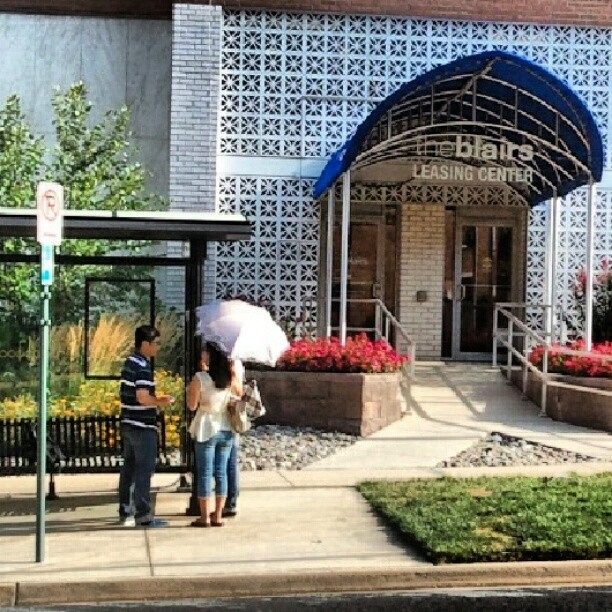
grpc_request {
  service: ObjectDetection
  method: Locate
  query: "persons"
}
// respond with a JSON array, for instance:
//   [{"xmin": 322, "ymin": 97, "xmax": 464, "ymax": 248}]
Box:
[
  {"xmin": 183, "ymin": 343, "xmax": 242, "ymax": 529},
  {"xmin": 209, "ymin": 360, "xmax": 242, "ymax": 517},
  {"xmin": 186, "ymin": 344, "xmax": 213, "ymax": 513},
  {"xmin": 115, "ymin": 324, "xmax": 173, "ymax": 530}
]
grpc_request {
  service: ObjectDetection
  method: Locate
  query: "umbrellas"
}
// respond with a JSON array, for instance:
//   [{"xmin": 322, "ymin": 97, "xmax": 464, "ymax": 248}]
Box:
[{"xmin": 193, "ymin": 298, "xmax": 291, "ymax": 368}]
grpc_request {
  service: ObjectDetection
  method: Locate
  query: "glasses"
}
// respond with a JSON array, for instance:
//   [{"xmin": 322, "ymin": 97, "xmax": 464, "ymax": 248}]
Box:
[{"xmin": 150, "ymin": 341, "xmax": 161, "ymax": 345}]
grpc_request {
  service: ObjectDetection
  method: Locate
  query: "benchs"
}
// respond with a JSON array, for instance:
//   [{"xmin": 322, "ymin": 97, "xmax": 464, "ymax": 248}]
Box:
[{"xmin": 0, "ymin": 416, "xmax": 190, "ymax": 500}]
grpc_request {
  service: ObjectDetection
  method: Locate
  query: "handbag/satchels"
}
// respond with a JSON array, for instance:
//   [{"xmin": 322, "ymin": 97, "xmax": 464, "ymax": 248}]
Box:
[
  {"xmin": 229, "ymin": 401, "xmax": 249, "ymax": 433},
  {"xmin": 242, "ymin": 380, "xmax": 266, "ymax": 421}
]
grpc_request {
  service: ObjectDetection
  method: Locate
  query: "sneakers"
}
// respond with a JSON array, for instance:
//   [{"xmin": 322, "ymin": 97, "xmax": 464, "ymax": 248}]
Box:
[
  {"xmin": 137, "ymin": 520, "xmax": 167, "ymax": 528},
  {"xmin": 121, "ymin": 516, "xmax": 135, "ymax": 526}
]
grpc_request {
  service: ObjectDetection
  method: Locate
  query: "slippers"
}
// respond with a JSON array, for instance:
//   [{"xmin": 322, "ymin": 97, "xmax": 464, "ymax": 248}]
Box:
[
  {"xmin": 210, "ymin": 510, "xmax": 236, "ymax": 516},
  {"xmin": 211, "ymin": 523, "xmax": 223, "ymax": 526},
  {"xmin": 192, "ymin": 518, "xmax": 210, "ymax": 527}
]
